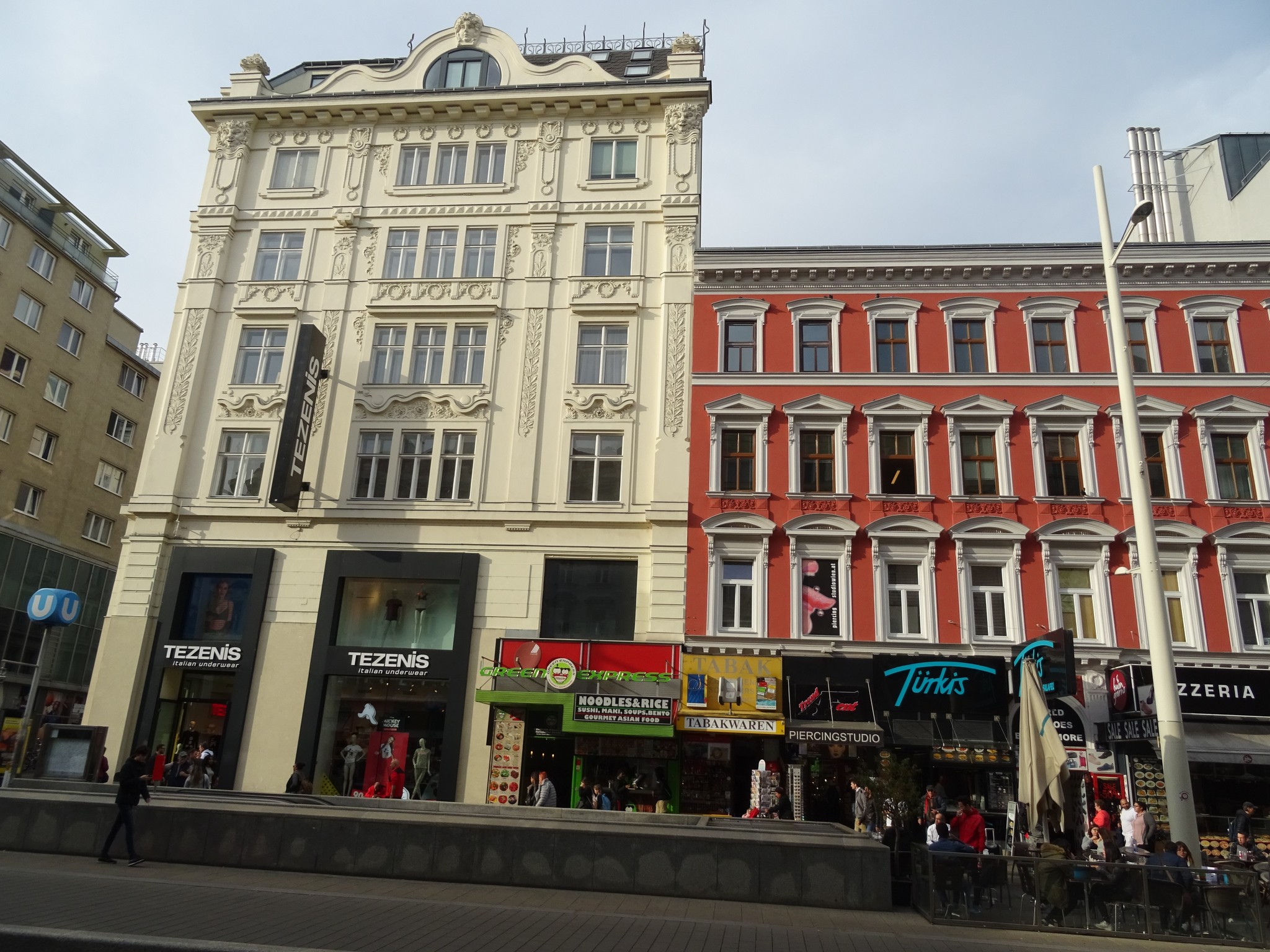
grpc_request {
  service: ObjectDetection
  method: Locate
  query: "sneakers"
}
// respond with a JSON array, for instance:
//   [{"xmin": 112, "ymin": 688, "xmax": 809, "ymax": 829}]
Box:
[
  {"xmin": 1104, "ymin": 923, "xmax": 1116, "ymax": 931},
  {"xmin": 97, "ymin": 857, "xmax": 117, "ymax": 864},
  {"xmin": 128, "ymin": 858, "xmax": 146, "ymax": 866},
  {"xmin": 1095, "ymin": 921, "xmax": 1107, "ymax": 929}
]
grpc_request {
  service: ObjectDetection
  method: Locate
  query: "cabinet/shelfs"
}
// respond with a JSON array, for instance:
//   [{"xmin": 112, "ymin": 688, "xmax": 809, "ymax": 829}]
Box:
[{"xmin": 749, "ymin": 759, "xmax": 805, "ymax": 821}]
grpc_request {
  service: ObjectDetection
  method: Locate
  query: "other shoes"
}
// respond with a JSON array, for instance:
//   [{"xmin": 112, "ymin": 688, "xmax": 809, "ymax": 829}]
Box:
[
  {"xmin": 1042, "ymin": 917, "xmax": 1062, "ymax": 927},
  {"xmin": 1169, "ymin": 926, "xmax": 1194, "ymax": 936}
]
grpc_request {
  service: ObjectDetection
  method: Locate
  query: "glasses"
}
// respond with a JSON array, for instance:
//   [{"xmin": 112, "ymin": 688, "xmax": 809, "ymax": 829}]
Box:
[
  {"xmin": 1092, "ymin": 829, "xmax": 1099, "ymax": 831},
  {"xmin": 927, "ymin": 790, "xmax": 934, "ymax": 792},
  {"xmin": 161, "ymin": 748, "xmax": 165, "ymax": 750},
  {"xmin": 1133, "ymin": 806, "xmax": 1140, "ymax": 808},
  {"xmin": 1178, "ymin": 849, "xmax": 1187, "ymax": 854},
  {"xmin": 786, "ymin": 771, "xmax": 804, "ymax": 821},
  {"xmin": 293, "ymin": 765, "xmax": 295, "ymax": 767}
]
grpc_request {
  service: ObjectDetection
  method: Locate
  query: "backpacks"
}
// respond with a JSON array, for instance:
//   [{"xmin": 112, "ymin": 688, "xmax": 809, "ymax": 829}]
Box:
[
  {"xmin": 586, "ymin": 785, "xmax": 597, "ymax": 809},
  {"xmin": 1229, "ymin": 815, "xmax": 1242, "ymax": 842}
]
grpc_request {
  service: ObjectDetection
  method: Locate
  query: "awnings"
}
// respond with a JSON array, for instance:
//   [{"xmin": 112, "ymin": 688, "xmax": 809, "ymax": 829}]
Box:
[{"xmin": 677, "ymin": 711, "xmax": 884, "ymax": 745}]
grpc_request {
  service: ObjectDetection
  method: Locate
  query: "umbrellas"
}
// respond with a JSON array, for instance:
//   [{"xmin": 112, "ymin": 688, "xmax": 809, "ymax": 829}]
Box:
[{"xmin": 1019, "ymin": 656, "xmax": 1070, "ymax": 842}]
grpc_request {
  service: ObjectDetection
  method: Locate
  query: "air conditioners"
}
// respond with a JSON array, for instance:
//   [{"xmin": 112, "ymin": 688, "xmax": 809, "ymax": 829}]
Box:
[{"xmin": 708, "ymin": 743, "xmax": 730, "ymax": 760}]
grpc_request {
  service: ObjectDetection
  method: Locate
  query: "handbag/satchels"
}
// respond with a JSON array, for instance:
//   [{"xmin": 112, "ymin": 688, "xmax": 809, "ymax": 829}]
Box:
[
  {"xmin": 102, "ymin": 772, "xmax": 109, "ymax": 783},
  {"xmin": 1155, "ymin": 828, "xmax": 1164, "ymax": 841}
]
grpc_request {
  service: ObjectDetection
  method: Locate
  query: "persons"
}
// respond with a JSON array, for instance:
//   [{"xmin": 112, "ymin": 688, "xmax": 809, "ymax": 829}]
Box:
[
  {"xmin": 339, "ymin": 734, "xmax": 368, "ymax": 796},
  {"xmin": 526, "ymin": 771, "xmax": 672, "ymax": 814},
  {"xmin": 378, "ymin": 587, "xmax": 437, "ymax": 647},
  {"xmin": 365, "ymin": 759, "xmax": 404, "ymax": 801},
  {"xmin": 97, "ymin": 740, "xmax": 222, "ymax": 867},
  {"xmin": 285, "ymin": 762, "xmax": 305, "ymax": 796},
  {"xmin": 1020, "ymin": 797, "xmax": 1266, "ymax": 937},
  {"xmin": 377, "ymin": 735, "xmax": 397, "ymax": 794},
  {"xmin": 410, "ymin": 738, "xmax": 432, "ymax": 799},
  {"xmin": 40, "ymin": 700, "xmax": 61, "ymax": 727},
  {"xmin": 204, "ymin": 579, "xmax": 234, "ymax": 635},
  {"xmin": 171, "ymin": 721, "xmax": 200, "ymax": 761},
  {"xmin": 764, "ymin": 786, "xmax": 794, "ymax": 820},
  {"xmin": 852, "ymin": 778, "xmax": 988, "ymax": 913},
  {"xmin": 827, "ymin": 744, "xmax": 845, "ymax": 758}
]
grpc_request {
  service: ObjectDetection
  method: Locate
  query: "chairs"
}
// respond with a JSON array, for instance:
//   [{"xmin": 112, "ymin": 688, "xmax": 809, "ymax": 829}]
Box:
[{"xmin": 935, "ymin": 842, "xmax": 1270, "ymax": 942}]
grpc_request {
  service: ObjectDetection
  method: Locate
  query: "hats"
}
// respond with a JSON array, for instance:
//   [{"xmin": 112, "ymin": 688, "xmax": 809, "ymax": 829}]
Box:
[
  {"xmin": 179, "ymin": 752, "xmax": 187, "ymax": 756},
  {"xmin": 1243, "ymin": 802, "xmax": 1258, "ymax": 808},
  {"xmin": 926, "ymin": 785, "xmax": 934, "ymax": 790}
]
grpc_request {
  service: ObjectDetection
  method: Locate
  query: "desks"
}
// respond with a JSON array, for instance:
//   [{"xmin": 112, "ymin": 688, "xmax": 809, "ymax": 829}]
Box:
[
  {"xmin": 1066, "ymin": 875, "xmax": 1114, "ymax": 929},
  {"xmin": 1192, "ymin": 876, "xmax": 1225, "ymax": 937},
  {"xmin": 602, "ymin": 786, "xmax": 653, "ymax": 812}
]
